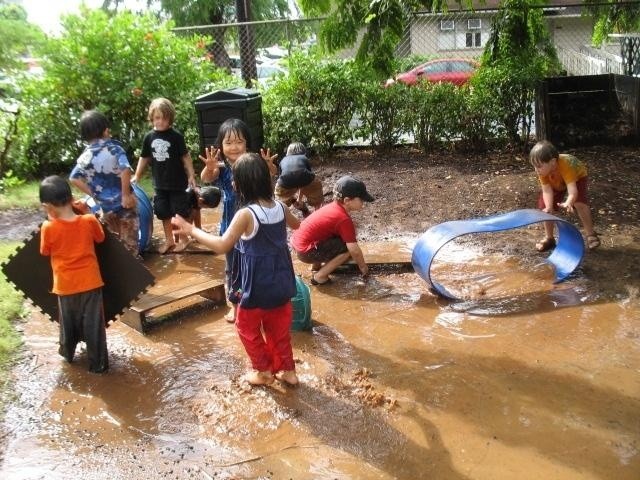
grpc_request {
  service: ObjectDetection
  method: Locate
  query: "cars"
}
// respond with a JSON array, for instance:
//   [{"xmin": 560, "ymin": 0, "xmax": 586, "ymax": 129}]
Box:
[
  {"xmin": 228, "ymin": 35, "xmax": 316, "ymax": 88},
  {"xmin": 383, "ymin": 56, "xmax": 483, "ymax": 89}
]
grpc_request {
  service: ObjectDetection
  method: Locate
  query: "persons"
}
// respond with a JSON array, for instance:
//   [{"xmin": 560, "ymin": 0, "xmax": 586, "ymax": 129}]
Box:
[
  {"xmin": 274, "ymin": 140, "xmax": 323, "ymax": 210},
  {"xmin": 290, "ymin": 174, "xmax": 375, "ymax": 287},
  {"xmin": 198, "ymin": 118, "xmax": 280, "ymax": 324},
  {"xmin": 151, "ymin": 185, "xmax": 221, "ymax": 244},
  {"xmin": 38, "ymin": 174, "xmax": 109, "ymax": 374},
  {"xmin": 527, "ymin": 139, "xmax": 600, "ymax": 251},
  {"xmin": 130, "ymin": 97, "xmax": 198, "ymax": 254},
  {"xmin": 68, "ymin": 108, "xmax": 144, "ymax": 262},
  {"xmin": 170, "ymin": 152, "xmax": 301, "ymax": 388}
]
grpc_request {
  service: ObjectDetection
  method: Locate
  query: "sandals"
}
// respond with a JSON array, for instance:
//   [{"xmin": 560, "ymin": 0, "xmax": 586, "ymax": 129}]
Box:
[
  {"xmin": 586, "ymin": 231, "xmax": 600, "ymax": 249},
  {"xmin": 536, "ymin": 237, "xmax": 555, "ymax": 252},
  {"xmin": 311, "ymin": 273, "xmax": 334, "ymax": 285}
]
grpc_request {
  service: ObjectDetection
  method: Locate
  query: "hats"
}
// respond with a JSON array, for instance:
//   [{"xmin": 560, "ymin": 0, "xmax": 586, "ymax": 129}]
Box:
[{"xmin": 334, "ymin": 176, "xmax": 374, "ymax": 202}]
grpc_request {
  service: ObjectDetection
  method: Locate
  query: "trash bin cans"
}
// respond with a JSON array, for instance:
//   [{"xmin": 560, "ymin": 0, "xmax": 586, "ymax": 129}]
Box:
[{"xmin": 194, "ymin": 88, "xmax": 264, "ymax": 166}]
